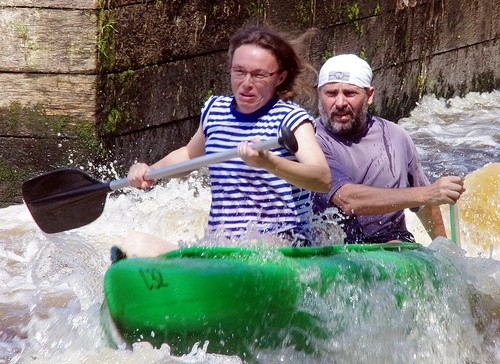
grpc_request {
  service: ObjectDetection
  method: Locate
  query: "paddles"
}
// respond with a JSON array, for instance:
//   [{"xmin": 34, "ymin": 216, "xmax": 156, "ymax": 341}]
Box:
[
  {"xmin": 449, "ymin": 204, "xmax": 461, "ymax": 248},
  {"xmin": 21, "ymin": 125, "xmax": 299, "ymax": 235}
]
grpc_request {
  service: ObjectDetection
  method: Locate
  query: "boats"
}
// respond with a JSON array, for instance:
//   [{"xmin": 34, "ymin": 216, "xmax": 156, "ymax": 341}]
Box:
[{"xmin": 102, "ymin": 241, "xmax": 499, "ymax": 364}]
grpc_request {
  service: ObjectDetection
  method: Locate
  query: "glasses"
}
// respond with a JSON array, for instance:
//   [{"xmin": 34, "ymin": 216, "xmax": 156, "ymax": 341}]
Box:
[{"xmin": 229, "ymin": 66, "xmax": 279, "ymax": 83}]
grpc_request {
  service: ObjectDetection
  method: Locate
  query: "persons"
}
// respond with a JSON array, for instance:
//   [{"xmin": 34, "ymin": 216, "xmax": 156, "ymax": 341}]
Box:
[
  {"xmin": 313, "ymin": 53, "xmax": 468, "ymax": 248},
  {"xmin": 127, "ymin": 23, "xmax": 334, "ymax": 246}
]
